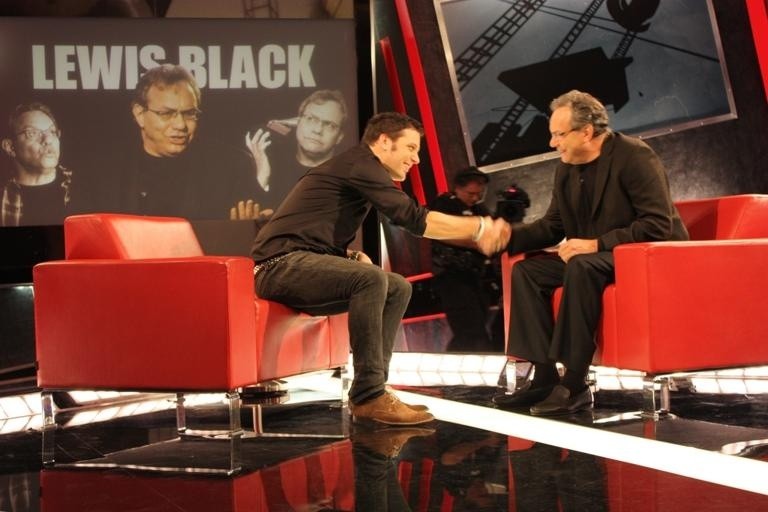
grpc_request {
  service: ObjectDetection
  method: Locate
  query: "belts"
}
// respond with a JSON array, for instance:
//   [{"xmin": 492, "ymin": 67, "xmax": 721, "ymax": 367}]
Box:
[{"xmin": 253, "ymin": 255, "xmax": 283, "ymax": 275}]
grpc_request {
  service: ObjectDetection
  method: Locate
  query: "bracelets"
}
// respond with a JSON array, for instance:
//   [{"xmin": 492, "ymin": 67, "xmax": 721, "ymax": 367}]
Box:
[{"xmin": 472, "ymin": 217, "xmax": 484, "ymax": 243}]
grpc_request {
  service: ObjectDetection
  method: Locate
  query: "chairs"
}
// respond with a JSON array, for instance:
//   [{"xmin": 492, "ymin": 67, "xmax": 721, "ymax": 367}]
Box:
[
  {"xmin": 25, "ymin": 211, "xmax": 358, "ymax": 477},
  {"xmin": 498, "ymin": 190, "xmax": 765, "ymax": 422}
]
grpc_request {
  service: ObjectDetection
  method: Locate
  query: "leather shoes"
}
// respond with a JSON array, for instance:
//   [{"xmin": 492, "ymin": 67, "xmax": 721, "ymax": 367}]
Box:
[
  {"xmin": 348, "ymin": 392, "xmax": 434, "ymax": 426},
  {"xmin": 493, "ymin": 380, "xmax": 553, "ymax": 406},
  {"xmin": 349, "ymin": 427, "xmax": 436, "ymax": 458},
  {"xmin": 530, "ymin": 386, "xmax": 594, "ymax": 416}
]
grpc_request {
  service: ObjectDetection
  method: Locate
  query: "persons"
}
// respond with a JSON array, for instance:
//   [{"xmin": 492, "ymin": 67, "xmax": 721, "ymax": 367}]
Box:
[
  {"xmin": 508, "ymin": 412, "xmax": 607, "ymax": 511},
  {"xmin": 434, "ymin": 383, "xmax": 507, "ymax": 511},
  {"xmin": 76, "ymin": 63, "xmax": 273, "ymax": 219},
  {"xmin": 429, "ymin": 168, "xmax": 496, "ymax": 350},
  {"xmin": 346, "ymin": 411, "xmax": 438, "ymax": 511},
  {"xmin": 0, "ymin": 101, "xmax": 75, "ymax": 225},
  {"xmin": 246, "ymin": 89, "xmax": 347, "ymax": 209},
  {"xmin": 248, "ymin": 112, "xmax": 506, "ymax": 427},
  {"xmin": 489, "ymin": 90, "xmax": 691, "ymax": 416}
]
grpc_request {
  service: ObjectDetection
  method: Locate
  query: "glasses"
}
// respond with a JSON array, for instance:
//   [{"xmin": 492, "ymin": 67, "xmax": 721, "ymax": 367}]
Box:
[
  {"xmin": 146, "ymin": 108, "xmax": 203, "ymax": 120},
  {"xmin": 9, "ymin": 128, "xmax": 61, "ymax": 140}
]
